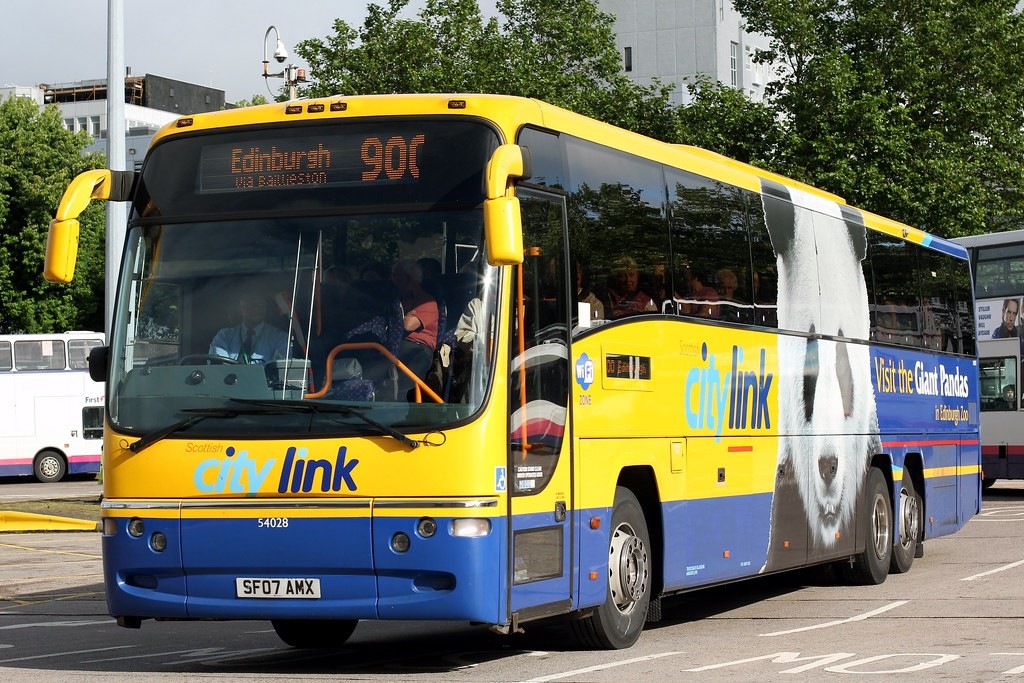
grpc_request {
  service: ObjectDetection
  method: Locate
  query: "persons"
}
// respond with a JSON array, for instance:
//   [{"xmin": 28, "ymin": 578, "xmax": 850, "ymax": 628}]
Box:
[
  {"xmin": 207, "ymin": 280, "xmax": 293, "ymax": 364},
  {"xmin": 453, "ymin": 294, "xmax": 495, "ymax": 354},
  {"xmin": 999, "ymin": 385, "xmax": 1016, "ymax": 409},
  {"xmin": 675, "ymin": 267, "xmax": 720, "ymax": 319},
  {"xmin": 375, "ymin": 259, "xmax": 438, "ymax": 401},
  {"xmin": 575, "ymin": 261, "xmax": 604, "ymax": 320},
  {"xmin": 604, "ymin": 256, "xmax": 657, "ymax": 318},
  {"xmin": 991, "ymin": 299, "xmax": 1019, "ymax": 339},
  {"xmin": 713, "ymin": 268, "xmax": 738, "ymax": 300}
]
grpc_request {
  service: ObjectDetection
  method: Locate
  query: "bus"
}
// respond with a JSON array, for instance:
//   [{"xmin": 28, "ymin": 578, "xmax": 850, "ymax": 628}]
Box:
[
  {"xmin": 45, "ymin": 91, "xmax": 984, "ymax": 650},
  {"xmin": 0, "ymin": 331, "xmax": 106, "ymax": 483},
  {"xmin": 949, "ymin": 229, "xmax": 1024, "ymax": 487}
]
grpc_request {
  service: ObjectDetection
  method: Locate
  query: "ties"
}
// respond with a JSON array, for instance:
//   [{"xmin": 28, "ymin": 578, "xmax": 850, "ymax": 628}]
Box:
[{"xmin": 236, "ymin": 329, "xmax": 255, "ymax": 365}]
